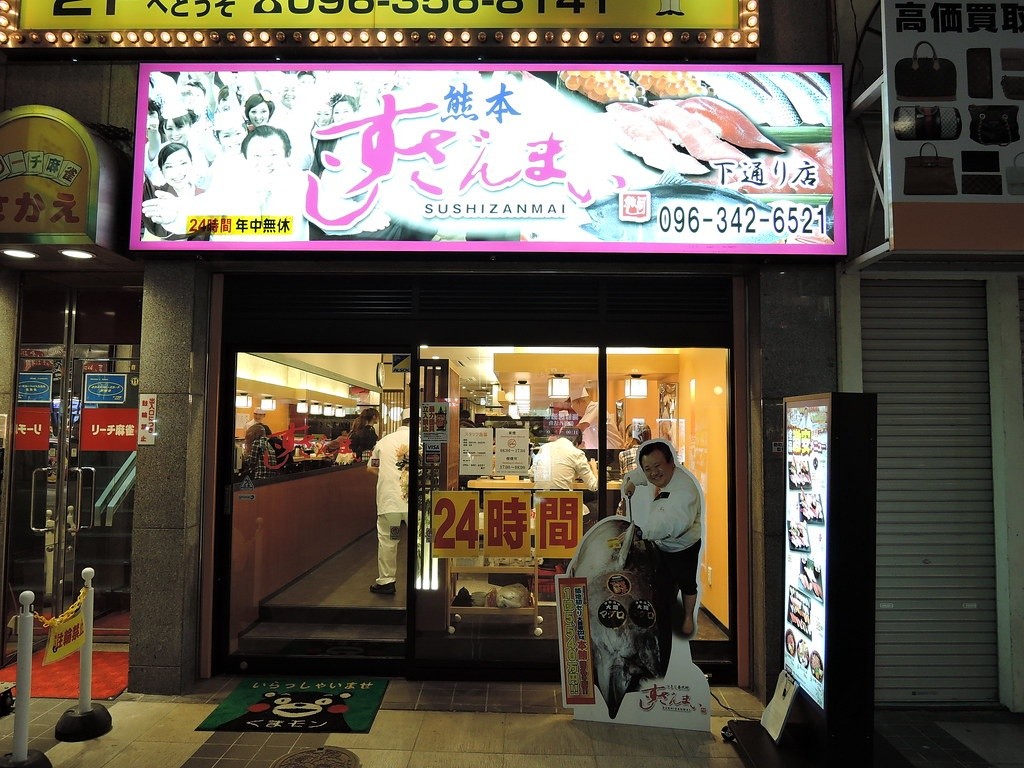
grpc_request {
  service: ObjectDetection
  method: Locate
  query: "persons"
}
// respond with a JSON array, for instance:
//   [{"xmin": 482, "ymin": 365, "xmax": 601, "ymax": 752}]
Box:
[
  {"xmin": 323, "ymin": 430, "xmax": 360, "ymax": 466},
  {"xmin": 547, "ymin": 384, "xmax": 625, "ymax": 465},
  {"xmin": 527, "ymin": 427, "xmax": 599, "ymax": 539},
  {"xmin": 144, "ymin": 70, "xmax": 359, "ymax": 242},
  {"xmin": 243, "ymin": 407, "xmax": 293, "ymax": 479},
  {"xmin": 350, "ymin": 408, "xmax": 380, "ymax": 457},
  {"xmin": 625, "ymin": 442, "xmax": 702, "ymax": 637},
  {"xmin": 367, "ymin": 407, "xmax": 434, "ymax": 595},
  {"xmin": 459, "ymin": 410, "xmax": 477, "ymax": 428},
  {"xmin": 616, "ymin": 423, "xmax": 652, "ymax": 516}
]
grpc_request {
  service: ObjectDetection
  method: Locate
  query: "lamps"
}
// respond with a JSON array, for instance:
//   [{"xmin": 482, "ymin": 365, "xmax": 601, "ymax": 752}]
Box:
[
  {"xmin": 296, "ymin": 400, "xmax": 309, "ymax": 413},
  {"xmin": 513, "ymin": 380, "xmax": 536, "ymax": 400},
  {"xmin": 261, "ymin": 396, "xmax": 276, "ymax": 411},
  {"xmin": 310, "ymin": 402, "xmax": 322, "ymax": 415},
  {"xmin": 548, "ymin": 374, "xmax": 570, "ymax": 399},
  {"xmin": 236, "ymin": 393, "xmax": 252, "ymax": 408},
  {"xmin": 324, "ymin": 404, "xmax": 334, "ymax": 416},
  {"xmin": 625, "ymin": 374, "xmax": 647, "ymax": 398},
  {"xmin": 335, "ymin": 406, "xmax": 346, "ymax": 417}
]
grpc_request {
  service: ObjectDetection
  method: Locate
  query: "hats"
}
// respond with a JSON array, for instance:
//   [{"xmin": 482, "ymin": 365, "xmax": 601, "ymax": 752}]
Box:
[
  {"xmin": 254, "ymin": 407, "xmax": 265, "ymax": 414},
  {"xmin": 403, "ymin": 408, "xmax": 421, "ymax": 420},
  {"xmin": 570, "ymin": 384, "xmax": 588, "ymax": 401}
]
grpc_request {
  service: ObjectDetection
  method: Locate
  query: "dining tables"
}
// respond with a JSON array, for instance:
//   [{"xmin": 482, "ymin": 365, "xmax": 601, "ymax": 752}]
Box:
[{"xmin": 468, "ymin": 475, "xmax": 623, "ymax": 490}]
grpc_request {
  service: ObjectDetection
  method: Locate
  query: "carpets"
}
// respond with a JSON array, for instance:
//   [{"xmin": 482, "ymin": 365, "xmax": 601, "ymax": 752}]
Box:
[
  {"xmin": 0, "ymin": 651, "xmax": 128, "ymax": 701},
  {"xmin": 189, "ymin": 672, "xmax": 391, "ymax": 735}
]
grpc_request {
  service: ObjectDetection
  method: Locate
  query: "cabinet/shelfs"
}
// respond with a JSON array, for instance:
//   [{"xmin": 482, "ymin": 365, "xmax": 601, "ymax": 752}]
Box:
[{"xmin": 445, "ymin": 528, "xmax": 544, "ymax": 637}]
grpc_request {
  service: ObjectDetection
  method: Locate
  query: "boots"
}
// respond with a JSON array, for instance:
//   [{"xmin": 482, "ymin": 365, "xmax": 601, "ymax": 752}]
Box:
[{"xmin": 680, "ymin": 591, "xmax": 697, "ymax": 636}]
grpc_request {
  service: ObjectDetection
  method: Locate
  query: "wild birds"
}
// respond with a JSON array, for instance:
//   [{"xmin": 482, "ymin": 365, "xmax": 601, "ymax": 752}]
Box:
[{"xmin": 566, "ymin": 518, "xmax": 673, "ymax": 720}]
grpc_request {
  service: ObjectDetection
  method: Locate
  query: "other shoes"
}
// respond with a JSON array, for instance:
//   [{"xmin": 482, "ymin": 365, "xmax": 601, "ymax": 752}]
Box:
[{"xmin": 370, "ymin": 580, "xmax": 396, "ymax": 594}]
